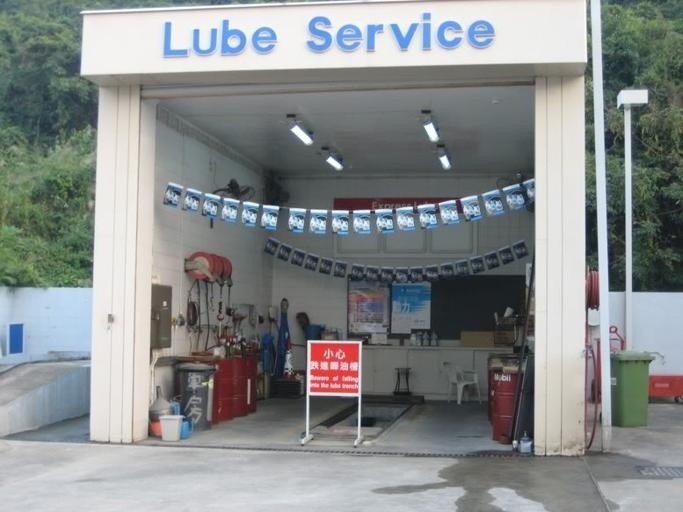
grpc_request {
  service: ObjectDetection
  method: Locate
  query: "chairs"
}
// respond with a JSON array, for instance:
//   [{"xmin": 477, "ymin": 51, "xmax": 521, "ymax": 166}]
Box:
[{"xmin": 442, "ymin": 362, "xmax": 481, "ymax": 405}]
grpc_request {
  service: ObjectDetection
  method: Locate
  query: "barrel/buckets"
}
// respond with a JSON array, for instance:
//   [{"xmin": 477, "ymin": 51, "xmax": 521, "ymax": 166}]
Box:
[
  {"xmin": 232, "ymin": 355, "xmax": 246, "ymax": 417},
  {"xmin": 491, "ymin": 367, "xmax": 522, "ymax": 441},
  {"xmin": 215, "ymin": 359, "xmax": 232, "ymax": 424},
  {"xmin": 180, "ymin": 421, "xmax": 194, "ymax": 438},
  {"xmin": 245, "ymin": 357, "xmax": 257, "ymax": 412}
]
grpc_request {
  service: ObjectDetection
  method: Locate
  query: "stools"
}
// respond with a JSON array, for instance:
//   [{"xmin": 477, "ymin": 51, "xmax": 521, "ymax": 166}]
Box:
[{"xmin": 393, "ymin": 368, "xmax": 411, "ymax": 396}]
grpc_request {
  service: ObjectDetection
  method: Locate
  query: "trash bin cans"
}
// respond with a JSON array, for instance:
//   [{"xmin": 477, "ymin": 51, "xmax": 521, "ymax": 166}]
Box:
[
  {"xmin": 170, "ymin": 394, "xmax": 183, "ymax": 415},
  {"xmin": 612, "ymin": 350, "xmax": 656, "ymax": 428},
  {"xmin": 158, "ymin": 414, "xmax": 183, "ymax": 442}
]
